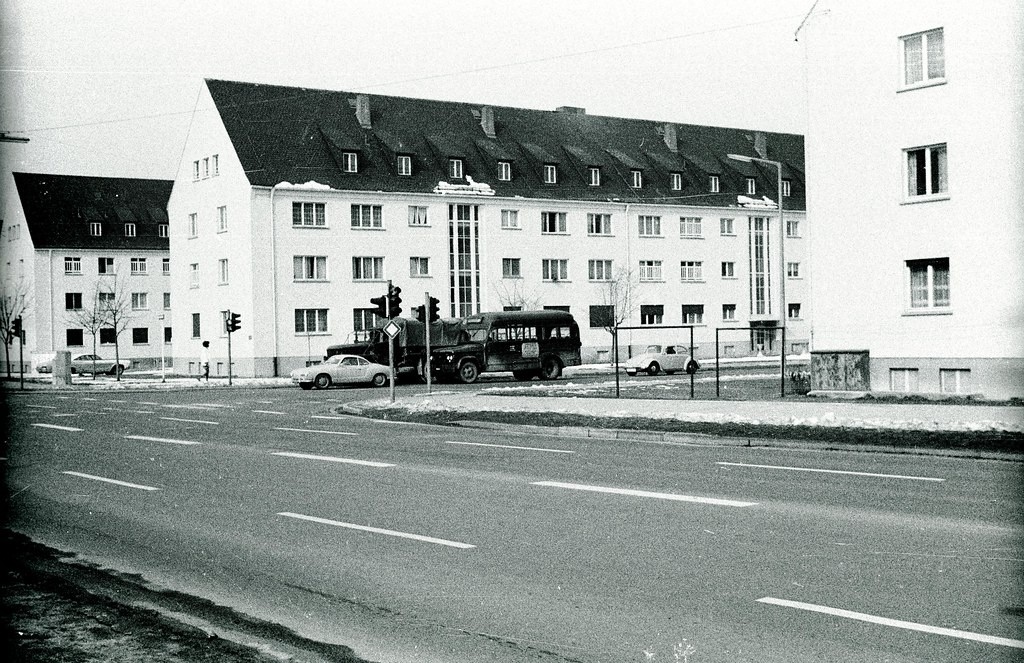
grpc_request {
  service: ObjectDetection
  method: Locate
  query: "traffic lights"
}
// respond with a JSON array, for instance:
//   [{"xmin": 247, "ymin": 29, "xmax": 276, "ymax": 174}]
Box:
[
  {"xmin": 387, "ymin": 284, "xmax": 402, "ymax": 318},
  {"xmin": 371, "ymin": 295, "xmax": 390, "ymax": 318},
  {"xmin": 415, "ymin": 305, "xmax": 426, "ymax": 323},
  {"xmin": 11, "ymin": 318, "xmax": 20, "ymax": 337},
  {"xmin": 230, "ymin": 312, "xmax": 241, "ymax": 332},
  {"xmin": 429, "ymin": 296, "xmax": 440, "ymax": 323}
]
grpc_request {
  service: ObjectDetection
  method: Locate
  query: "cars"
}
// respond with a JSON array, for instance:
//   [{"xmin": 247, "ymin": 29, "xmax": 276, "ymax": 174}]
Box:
[
  {"xmin": 626, "ymin": 342, "xmax": 700, "ymax": 376},
  {"xmin": 291, "ymin": 353, "xmax": 398, "ymax": 390},
  {"xmin": 70, "ymin": 353, "xmax": 133, "ymax": 376},
  {"xmin": 36, "ymin": 359, "xmax": 54, "ymax": 373}
]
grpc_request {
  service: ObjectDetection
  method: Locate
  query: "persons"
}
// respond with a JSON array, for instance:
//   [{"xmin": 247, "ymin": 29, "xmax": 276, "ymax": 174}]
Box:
[{"xmin": 196, "ymin": 341, "xmax": 210, "ymax": 381}]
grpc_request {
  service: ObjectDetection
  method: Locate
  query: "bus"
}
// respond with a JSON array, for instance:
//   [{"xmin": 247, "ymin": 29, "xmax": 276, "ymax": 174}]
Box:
[{"xmin": 431, "ymin": 309, "xmax": 582, "ymax": 385}]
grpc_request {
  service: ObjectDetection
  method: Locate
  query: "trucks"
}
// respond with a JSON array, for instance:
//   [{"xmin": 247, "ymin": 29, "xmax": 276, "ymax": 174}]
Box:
[{"xmin": 322, "ymin": 315, "xmax": 471, "ymax": 371}]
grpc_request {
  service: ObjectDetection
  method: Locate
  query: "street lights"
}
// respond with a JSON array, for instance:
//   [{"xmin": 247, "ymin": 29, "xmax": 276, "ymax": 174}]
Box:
[{"xmin": 727, "ymin": 152, "xmax": 788, "ymax": 361}]
